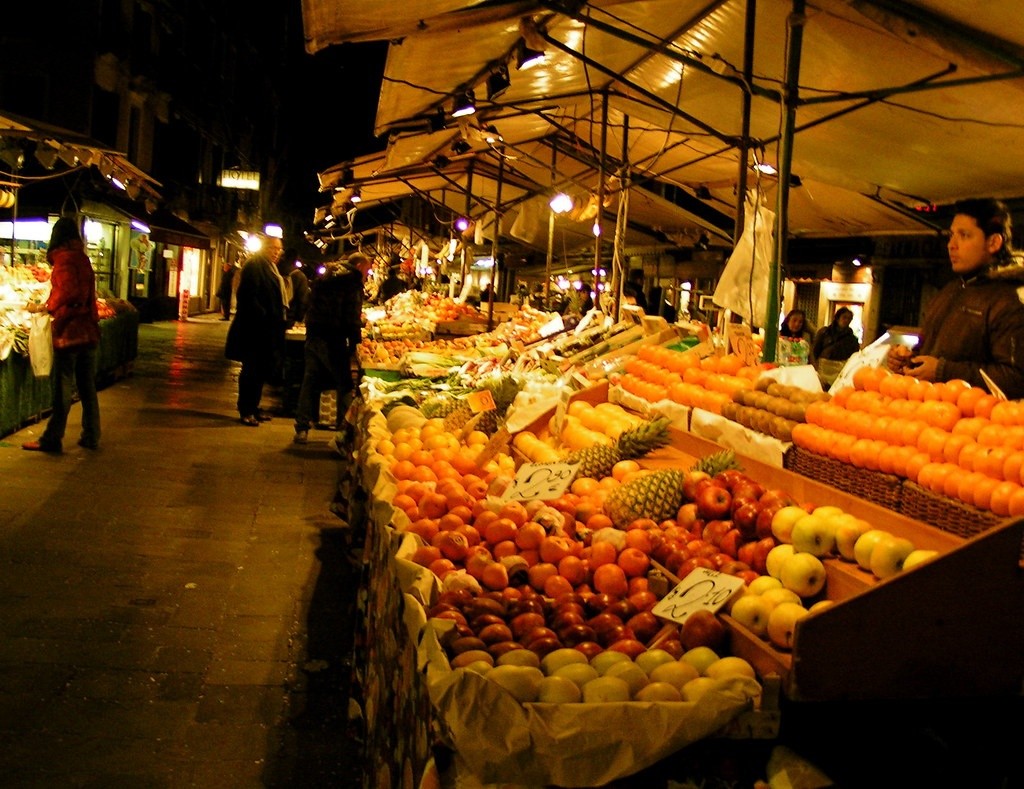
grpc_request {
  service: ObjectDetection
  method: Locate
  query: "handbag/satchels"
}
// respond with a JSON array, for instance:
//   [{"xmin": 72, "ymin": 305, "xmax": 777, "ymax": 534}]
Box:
[{"xmin": 29, "ymin": 311, "xmax": 55, "ymax": 377}]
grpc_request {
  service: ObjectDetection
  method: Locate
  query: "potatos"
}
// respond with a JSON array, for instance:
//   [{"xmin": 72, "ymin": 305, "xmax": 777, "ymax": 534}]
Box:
[{"xmin": 29, "ymin": 266, "xmax": 52, "ymax": 282}]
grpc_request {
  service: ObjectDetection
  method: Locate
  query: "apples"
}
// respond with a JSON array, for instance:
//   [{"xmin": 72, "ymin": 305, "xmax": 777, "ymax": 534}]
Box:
[
  {"xmin": 355, "ymin": 338, "xmax": 473, "ymax": 357},
  {"xmin": 378, "ymin": 298, "xmax": 487, "ymax": 338},
  {"xmin": 427, "ymin": 467, "xmax": 938, "ymax": 703}
]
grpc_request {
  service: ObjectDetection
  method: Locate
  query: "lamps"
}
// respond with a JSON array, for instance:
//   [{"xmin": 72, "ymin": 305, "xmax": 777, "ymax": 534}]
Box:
[
  {"xmin": 57, "ymin": 145, "xmax": 81, "ymax": 168},
  {"xmin": 788, "ymin": 173, "xmax": 802, "ymax": 189},
  {"xmin": 454, "ymin": 141, "xmax": 473, "ymax": 156},
  {"xmin": 432, "ymin": 155, "xmax": 452, "ymax": 169},
  {"xmin": 452, "ymin": 93, "xmax": 475, "ymax": 118},
  {"xmin": 0, "ymin": 146, "xmax": 25, "ymax": 170},
  {"xmin": 126, "ymin": 184, "xmax": 141, "ymax": 199},
  {"xmin": 516, "ymin": 37, "xmax": 545, "ymax": 71},
  {"xmin": 35, "ymin": 141, "xmax": 57, "ymax": 171},
  {"xmin": 486, "ymin": 71, "xmax": 510, "ymax": 101},
  {"xmin": 145, "ymin": 199, "xmax": 158, "ymax": 214},
  {"xmin": 111, "ymin": 170, "xmax": 127, "ymax": 189},
  {"xmin": 431, "ymin": 110, "xmax": 458, "ymax": 134},
  {"xmin": 697, "ymin": 186, "xmax": 711, "ymax": 201},
  {"xmin": 98, "ymin": 158, "xmax": 114, "ymax": 179},
  {"xmin": 76, "ymin": 149, "xmax": 94, "ymax": 168},
  {"xmin": 334, "ymin": 179, "xmax": 346, "ymax": 192}
]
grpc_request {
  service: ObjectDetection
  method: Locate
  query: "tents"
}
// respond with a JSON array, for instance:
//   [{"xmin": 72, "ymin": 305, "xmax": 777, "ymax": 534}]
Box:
[{"xmin": 284, "ymin": 0, "xmax": 1024, "ymax": 256}]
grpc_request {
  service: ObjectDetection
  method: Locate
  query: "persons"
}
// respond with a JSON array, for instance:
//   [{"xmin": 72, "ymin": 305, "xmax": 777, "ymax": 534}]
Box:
[
  {"xmin": 778, "ymin": 307, "xmax": 859, "ymax": 393},
  {"xmin": 21, "ymin": 217, "xmax": 102, "ymax": 455},
  {"xmin": 215, "ymin": 233, "xmax": 409, "ymax": 443},
  {"xmin": 616, "ymin": 269, "xmax": 647, "ymax": 322},
  {"xmin": 888, "ymin": 196, "xmax": 1024, "ymax": 401},
  {"xmin": 481, "ymin": 283, "xmax": 594, "ymax": 318}
]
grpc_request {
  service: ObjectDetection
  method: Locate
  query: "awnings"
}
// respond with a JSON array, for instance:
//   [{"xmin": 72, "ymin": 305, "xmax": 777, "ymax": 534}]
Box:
[
  {"xmin": 150, "ymin": 209, "xmax": 210, "ymax": 250},
  {"xmin": 0, "ymin": 110, "xmax": 163, "ymax": 199}
]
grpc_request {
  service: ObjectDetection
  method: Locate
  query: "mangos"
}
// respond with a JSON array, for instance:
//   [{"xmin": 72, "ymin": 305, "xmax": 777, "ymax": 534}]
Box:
[{"xmin": 96, "ymin": 300, "xmax": 115, "ymax": 319}]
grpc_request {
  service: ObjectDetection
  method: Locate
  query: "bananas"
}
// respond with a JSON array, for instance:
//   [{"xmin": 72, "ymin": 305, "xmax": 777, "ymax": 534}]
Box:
[{"xmin": 0, "ymin": 188, "xmax": 15, "ymax": 208}]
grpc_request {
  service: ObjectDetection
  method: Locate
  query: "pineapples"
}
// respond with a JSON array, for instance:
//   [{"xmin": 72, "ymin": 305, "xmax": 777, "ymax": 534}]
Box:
[
  {"xmin": 559, "ymin": 412, "xmax": 672, "ymax": 480},
  {"xmin": 101, "ymin": 290, "xmax": 133, "ymax": 312},
  {"xmin": 419, "ymin": 377, "xmax": 519, "ymax": 437},
  {"xmin": 602, "ymin": 446, "xmax": 746, "ymax": 528}
]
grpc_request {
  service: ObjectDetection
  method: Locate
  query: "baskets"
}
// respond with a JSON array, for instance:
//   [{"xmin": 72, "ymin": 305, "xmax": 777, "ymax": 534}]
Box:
[
  {"xmin": 901, "ymin": 482, "xmax": 1008, "ymax": 538},
  {"xmin": 785, "ymin": 443, "xmax": 903, "ymax": 513}
]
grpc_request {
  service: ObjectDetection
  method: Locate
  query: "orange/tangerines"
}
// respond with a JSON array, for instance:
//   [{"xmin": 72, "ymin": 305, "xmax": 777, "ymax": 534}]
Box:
[
  {"xmin": 790, "ymin": 365, "xmax": 1024, "ymax": 519},
  {"xmin": 373, "ymin": 400, "xmax": 658, "ymax": 598},
  {"xmin": 619, "ymin": 343, "xmax": 768, "ymax": 417}
]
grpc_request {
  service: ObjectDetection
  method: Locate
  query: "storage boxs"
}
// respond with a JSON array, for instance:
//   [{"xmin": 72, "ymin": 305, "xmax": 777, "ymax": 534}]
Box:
[{"xmin": 350, "ymin": 369, "xmax": 787, "ymax": 789}]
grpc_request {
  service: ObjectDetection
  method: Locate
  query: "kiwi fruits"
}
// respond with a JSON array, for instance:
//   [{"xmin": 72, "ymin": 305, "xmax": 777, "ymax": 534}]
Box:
[{"xmin": 721, "ymin": 377, "xmax": 835, "ymax": 442}]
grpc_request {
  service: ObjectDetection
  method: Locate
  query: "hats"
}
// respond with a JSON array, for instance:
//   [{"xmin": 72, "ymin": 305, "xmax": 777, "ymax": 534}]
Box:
[
  {"xmin": 579, "ymin": 283, "xmax": 592, "ymax": 292},
  {"xmin": 627, "ymin": 268, "xmax": 647, "ymax": 278}
]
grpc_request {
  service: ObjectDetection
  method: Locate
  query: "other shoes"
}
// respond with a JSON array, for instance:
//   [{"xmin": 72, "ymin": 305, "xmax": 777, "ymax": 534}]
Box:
[
  {"xmin": 240, "ymin": 414, "xmax": 259, "ymax": 426},
  {"xmin": 22, "ymin": 440, "xmax": 63, "ymax": 452},
  {"xmin": 219, "ymin": 316, "xmax": 229, "ymax": 321},
  {"xmin": 293, "ymin": 430, "xmax": 307, "ymax": 443},
  {"xmin": 253, "ymin": 410, "xmax": 272, "ymax": 420},
  {"xmin": 77, "ymin": 438, "xmax": 97, "ymax": 450},
  {"xmin": 327, "ymin": 437, "xmax": 346, "ymax": 456}
]
